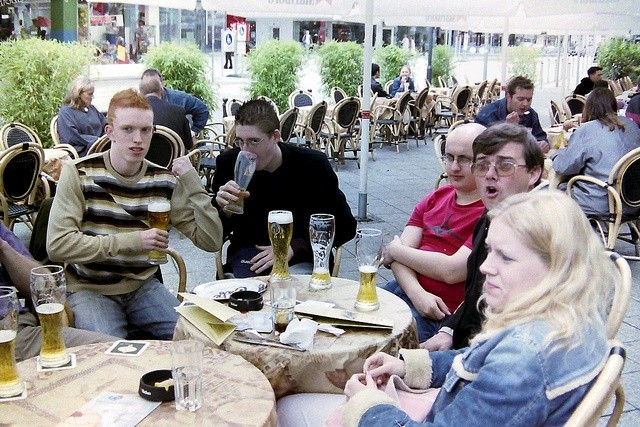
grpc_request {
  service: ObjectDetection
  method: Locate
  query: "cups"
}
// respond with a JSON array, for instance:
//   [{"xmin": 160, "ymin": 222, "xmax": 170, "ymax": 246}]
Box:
[
  {"xmin": 167, "ymin": 339, "xmax": 205, "ymax": 412},
  {"xmin": 29, "ymin": 263, "xmax": 70, "ymax": 367},
  {"xmin": 355, "ymin": 228, "xmax": 383, "ymax": 311},
  {"xmin": 0, "ymin": 285, "xmax": 25, "ymax": 397},
  {"xmin": 308, "ymin": 213, "xmax": 336, "ymax": 290},
  {"xmin": 146, "ymin": 196, "xmax": 171, "ymax": 265},
  {"xmin": 270, "ymin": 285, "xmax": 297, "ymax": 333},
  {"xmin": 223, "ymin": 150, "xmax": 258, "ymax": 215},
  {"xmin": 267, "ymin": 210, "xmax": 293, "ymax": 283}
]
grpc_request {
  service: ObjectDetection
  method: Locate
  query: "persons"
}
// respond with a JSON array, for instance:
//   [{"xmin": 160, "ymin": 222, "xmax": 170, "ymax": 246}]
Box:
[
  {"xmin": 303, "ymin": 30, "xmax": 313, "ymax": 53},
  {"xmin": 419, "ymin": 123, "xmax": 544, "ymax": 351},
  {"xmin": 143, "ymin": 69, "xmax": 208, "ymax": 148},
  {"xmin": 45, "ymin": 87, "xmax": 224, "ymax": 339},
  {"xmin": 400, "ymin": 34, "xmax": 416, "ymax": 50},
  {"xmin": 276, "ymin": 192, "xmax": 624, "ymax": 426},
  {"xmin": 550, "ymin": 88, "xmax": 639, "ymax": 217},
  {"xmin": 211, "ymin": 98, "xmax": 357, "ymax": 278},
  {"xmin": 384, "ymin": 124, "xmax": 487, "ymax": 342},
  {"xmin": 140, "ymin": 76, "xmax": 193, "ymax": 157},
  {"xmin": 56, "ymin": 77, "xmax": 107, "ymax": 157},
  {"xmin": 573, "ymin": 67, "xmax": 609, "ymax": 114},
  {"xmin": 474, "ymin": 75, "xmax": 548, "ymax": 155},
  {"xmin": 391, "ymin": 66, "xmax": 415, "ymax": 96},
  {"xmin": 372, "ymin": 63, "xmax": 389, "ymax": 99},
  {"xmin": 0, "ymin": 222, "xmax": 120, "ymax": 364}
]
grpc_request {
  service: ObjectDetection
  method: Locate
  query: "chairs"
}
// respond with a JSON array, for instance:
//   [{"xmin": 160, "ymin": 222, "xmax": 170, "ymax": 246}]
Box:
[
  {"xmin": 297, "ymin": 100, "xmax": 331, "ymax": 155},
  {"xmin": 602, "ymin": 251, "xmax": 631, "ymax": 339},
  {"xmin": 564, "ymin": 94, "xmax": 587, "ymax": 120},
  {"xmin": 0, "ymin": 142, "xmax": 46, "ymax": 229},
  {"xmin": 194, "ymin": 116, "xmax": 236, "ymax": 194},
  {"xmin": 485, "ymin": 75, "xmax": 501, "ymax": 103},
  {"xmin": 548, "ymin": 99, "xmax": 564, "ymax": 125},
  {"xmin": 450, "ymin": 75, "xmax": 461, "ymax": 86},
  {"xmin": 608, "ymin": 75, "xmax": 635, "ymax": 97},
  {"xmin": 425, "ymin": 78, "xmax": 434, "ymax": 90},
  {"xmin": 566, "ymin": 174, "xmax": 623, "ymax": 251},
  {"xmin": 587, "ymin": 146, "xmax": 640, "ymax": 259},
  {"xmin": 368, "ymin": 89, "xmax": 412, "ymax": 161},
  {"xmin": 564, "ymin": 341, "xmax": 629, "ymax": 426},
  {"xmin": 50, "ymin": 114, "xmax": 79, "ymax": 158},
  {"xmin": 196, "ymin": 122, "xmax": 226, "ymax": 153},
  {"xmin": 40, "ymin": 197, "xmax": 188, "ymax": 300},
  {"xmin": 279, "ymin": 107, "xmax": 300, "ymax": 142},
  {"xmin": 372, "ymin": 91, "xmax": 378, "ymax": 110},
  {"xmin": 433, "ymin": 86, "xmax": 472, "ymax": 134},
  {"xmin": 437, "ymin": 75, "xmax": 448, "ymax": 87},
  {"xmin": 384, "ymin": 79, "xmax": 396, "ymax": 95},
  {"xmin": 332, "ymin": 97, "xmax": 361, "ymax": 167},
  {"xmin": 0, "ymin": 122, "xmax": 43, "ymax": 153},
  {"xmin": 289, "ymin": 89, "xmax": 315, "ymax": 106},
  {"xmin": 87, "ymin": 125, "xmax": 201, "ymax": 176},
  {"xmin": 214, "ymin": 232, "xmax": 343, "ymax": 277},
  {"xmin": 331, "ymin": 86, "xmax": 346, "ymax": 103},
  {"xmin": 471, "ymin": 79, "xmax": 489, "ymax": 118},
  {"xmin": 226, "ymin": 98, "xmax": 242, "ymax": 117},
  {"xmin": 258, "ymin": 94, "xmax": 280, "ymax": 118},
  {"xmin": 401, "ymin": 87, "xmax": 430, "ymax": 148}
]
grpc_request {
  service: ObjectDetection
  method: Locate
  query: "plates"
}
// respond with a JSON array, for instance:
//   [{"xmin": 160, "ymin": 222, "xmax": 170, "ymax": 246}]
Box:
[{"xmin": 192, "ymin": 278, "xmax": 267, "ymax": 302}]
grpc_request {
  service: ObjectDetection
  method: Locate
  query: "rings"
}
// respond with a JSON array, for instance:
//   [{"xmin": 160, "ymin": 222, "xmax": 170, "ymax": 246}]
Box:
[{"xmin": 220, "ymin": 191, "xmax": 224, "ymax": 196}]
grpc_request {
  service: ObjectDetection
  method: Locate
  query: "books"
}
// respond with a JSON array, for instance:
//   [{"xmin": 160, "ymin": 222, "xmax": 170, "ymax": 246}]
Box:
[
  {"xmin": 174, "ymin": 290, "xmax": 237, "ymax": 347},
  {"xmin": 294, "ymin": 299, "xmax": 394, "ymax": 331}
]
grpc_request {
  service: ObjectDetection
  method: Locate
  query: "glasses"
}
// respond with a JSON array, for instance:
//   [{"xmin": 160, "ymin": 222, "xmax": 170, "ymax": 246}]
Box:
[
  {"xmin": 440, "ymin": 156, "xmax": 473, "ymax": 167},
  {"xmin": 471, "ymin": 161, "xmax": 530, "ymax": 176},
  {"xmin": 233, "ymin": 131, "xmax": 273, "ymax": 148}
]
